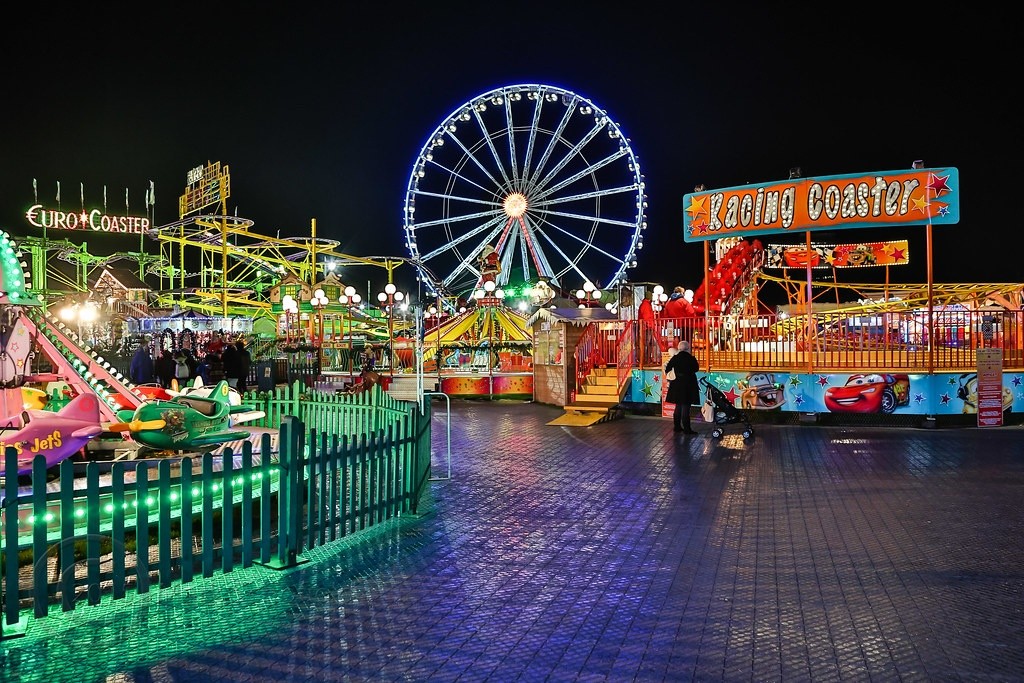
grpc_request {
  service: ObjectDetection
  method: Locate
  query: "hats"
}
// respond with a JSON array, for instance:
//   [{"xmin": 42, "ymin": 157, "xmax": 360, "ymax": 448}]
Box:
[
  {"xmin": 678, "ymin": 341, "xmax": 689, "ymax": 350},
  {"xmin": 646, "ymin": 291, "xmax": 653, "ymax": 302}
]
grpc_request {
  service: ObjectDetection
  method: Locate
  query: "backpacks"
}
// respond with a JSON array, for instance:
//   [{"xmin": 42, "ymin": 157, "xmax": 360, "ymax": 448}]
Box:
[{"xmin": 175, "ymin": 361, "xmax": 189, "ymax": 378}]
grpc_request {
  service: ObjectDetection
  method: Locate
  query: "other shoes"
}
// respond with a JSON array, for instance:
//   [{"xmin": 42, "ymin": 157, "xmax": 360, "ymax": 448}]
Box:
[
  {"xmin": 673, "ymin": 426, "xmax": 684, "ymax": 431},
  {"xmin": 684, "ymin": 427, "xmax": 698, "ymax": 434}
]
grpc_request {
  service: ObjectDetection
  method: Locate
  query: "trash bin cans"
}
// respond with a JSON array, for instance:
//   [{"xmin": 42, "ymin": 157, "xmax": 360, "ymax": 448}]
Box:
[{"xmin": 254, "ymin": 357, "xmax": 278, "ymax": 397}]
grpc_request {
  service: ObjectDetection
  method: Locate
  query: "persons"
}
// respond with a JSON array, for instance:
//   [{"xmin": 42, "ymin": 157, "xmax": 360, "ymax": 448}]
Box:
[
  {"xmin": 638, "ymin": 285, "xmax": 699, "ymax": 364},
  {"xmin": 130, "ymin": 338, "xmax": 250, "ymax": 395},
  {"xmin": 664, "ymin": 341, "xmax": 700, "ymax": 434}
]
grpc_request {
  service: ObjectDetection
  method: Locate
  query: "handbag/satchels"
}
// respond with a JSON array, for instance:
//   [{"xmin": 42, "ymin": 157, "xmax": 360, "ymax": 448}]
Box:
[
  {"xmin": 701, "ymin": 387, "xmax": 714, "ymax": 422},
  {"xmin": 227, "ymin": 378, "xmax": 238, "ymax": 388}
]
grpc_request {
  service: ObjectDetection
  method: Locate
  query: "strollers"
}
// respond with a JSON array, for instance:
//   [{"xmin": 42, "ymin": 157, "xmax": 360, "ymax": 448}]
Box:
[{"xmin": 699, "ymin": 376, "xmax": 755, "ymax": 440}]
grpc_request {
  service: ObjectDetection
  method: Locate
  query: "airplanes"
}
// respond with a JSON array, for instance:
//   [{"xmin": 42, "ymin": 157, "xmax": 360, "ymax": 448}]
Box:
[
  {"xmin": 107, "ymin": 376, "xmax": 264, "ymax": 450},
  {"xmin": 0, "ymin": 393, "xmax": 102, "ymax": 477}
]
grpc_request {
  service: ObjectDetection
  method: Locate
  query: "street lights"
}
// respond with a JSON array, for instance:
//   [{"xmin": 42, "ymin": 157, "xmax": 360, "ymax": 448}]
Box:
[
  {"xmin": 282, "ymin": 295, "xmax": 298, "ymax": 345},
  {"xmin": 652, "ymin": 285, "xmax": 668, "ymax": 318},
  {"xmin": 339, "ymin": 286, "xmax": 361, "ymax": 375},
  {"xmin": 310, "ymin": 289, "xmax": 330, "ymax": 380},
  {"xmin": 578, "ymin": 282, "xmax": 601, "ymax": 308},
  {"xmin": 378, "ymin": 284, "xmax": 403, "ymax": 376},
  {"xmin": 475, "ymin": 281, "xmax": 504, "ymax": 375},
  {"xmin": 426, "ymin": 308, "xmax": 441, "ymax": 328},
  {"xmin": 60, "ymin": 304, "xmax": 98, "ymax": 374}
]
